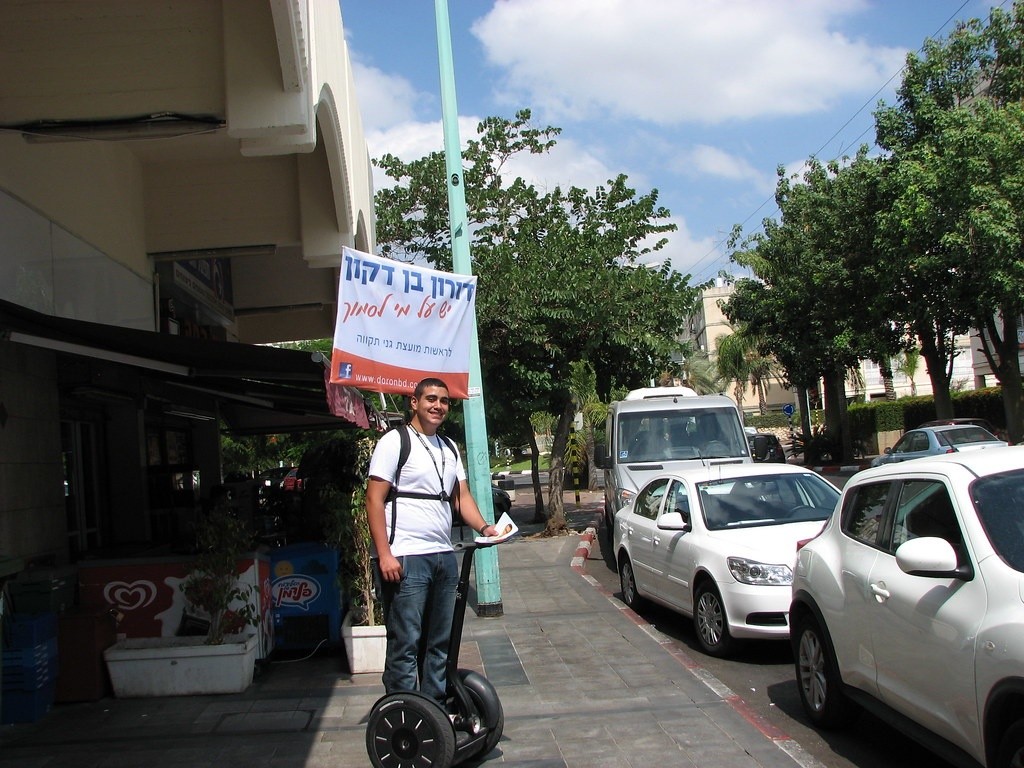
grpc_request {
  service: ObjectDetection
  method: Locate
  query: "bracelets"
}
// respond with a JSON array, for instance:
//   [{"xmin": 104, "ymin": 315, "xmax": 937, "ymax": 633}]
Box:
[{"xmin": 480, "ymin": 525, "xmax": 491, "ymax": 537}]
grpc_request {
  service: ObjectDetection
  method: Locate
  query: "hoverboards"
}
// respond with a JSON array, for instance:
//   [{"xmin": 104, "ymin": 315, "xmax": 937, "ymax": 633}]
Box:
[{"xmin": 365, "ymin": 535, "xmax": 511, "ymax": 768}]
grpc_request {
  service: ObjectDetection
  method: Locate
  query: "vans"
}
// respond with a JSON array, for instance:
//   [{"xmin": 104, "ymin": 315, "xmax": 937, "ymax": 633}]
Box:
[{"xmin": 591, "ymin": 386, "xmax": 755, "ymax": 547}]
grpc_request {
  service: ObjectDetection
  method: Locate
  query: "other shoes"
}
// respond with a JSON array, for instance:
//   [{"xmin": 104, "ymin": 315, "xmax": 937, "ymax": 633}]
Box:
[{"xmin": 448, "ymin": 714, "xmax": 463, "ymax": 724}]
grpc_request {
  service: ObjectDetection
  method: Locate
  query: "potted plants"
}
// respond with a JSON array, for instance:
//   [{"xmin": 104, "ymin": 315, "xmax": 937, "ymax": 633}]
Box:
[
  {"xmin": 104, "ymin": 501, "xmax": 259, "ymax": 693},
  {"xmin": 341, "ymin": 435, "xmax": 390, "ymax": 675}
]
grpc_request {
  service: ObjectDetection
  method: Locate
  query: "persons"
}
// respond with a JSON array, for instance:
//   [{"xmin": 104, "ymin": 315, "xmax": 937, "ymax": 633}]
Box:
[
  {"xmin": 366, "ymin": 379, "xmax": 499, "ymax": 704},
  {"xmin": 488, "ymin": 524, "xmax": 512, "ymax": 541}
]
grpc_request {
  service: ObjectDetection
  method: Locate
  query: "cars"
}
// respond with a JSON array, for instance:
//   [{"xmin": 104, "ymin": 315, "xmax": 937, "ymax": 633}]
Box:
[
  {"xmin": 870, "ymin": 425, "xmax": 1009, "ymax": 468},
  {"xmin": 250, "ymin": 466, "xmax": 301, "ymax": 496},
  {"xmin": 915, "ymin": 418, "xmax": 1002, "ymax": 441},
  {"xmin": 743, "ymin": 425, "xmax": 787, "ymax": 464},
  {"xmin": 787, "ymin": 444, "xmax": 1024, "ymax": 768},
  {"xmin": 612, "ymin": 463, "xmax": 849, "ymax": 654}
]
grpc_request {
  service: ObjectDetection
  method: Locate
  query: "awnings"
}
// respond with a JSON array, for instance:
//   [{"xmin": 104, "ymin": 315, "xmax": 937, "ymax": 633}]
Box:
[{"xmin": 0, "ymin": 312, "xmax": 369, "ymax": 433}]
